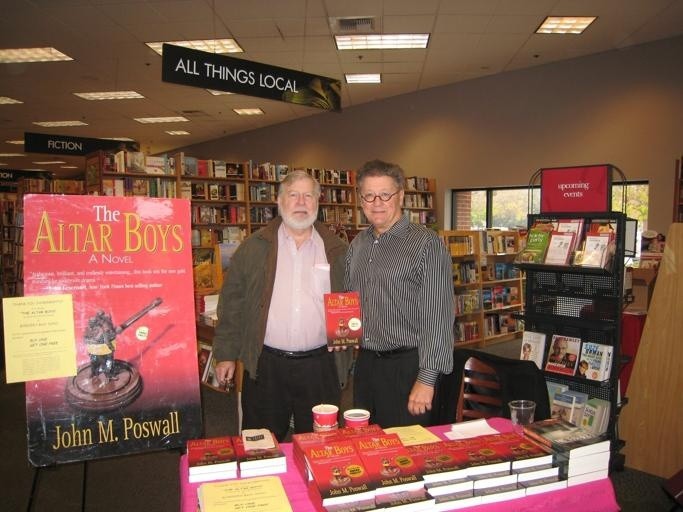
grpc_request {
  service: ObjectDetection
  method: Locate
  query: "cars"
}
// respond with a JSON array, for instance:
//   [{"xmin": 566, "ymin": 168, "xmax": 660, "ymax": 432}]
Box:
[
  {"xmin": 507, "ymin": 400, "xmax": 537, "ymax": 426},
  {"xmin": 311, "ymin": 404, "xmax": 340, "ymax": 427},
  {"xmin": 342, "ymin": 408, "xmax": 372, "ymax": 431}
]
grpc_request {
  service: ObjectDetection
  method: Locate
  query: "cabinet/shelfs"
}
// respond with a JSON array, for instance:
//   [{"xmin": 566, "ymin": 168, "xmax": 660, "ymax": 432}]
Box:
[
  {"xmin": 510, "ymin": 212, "xmax": 626, "ymax": 476},
  {"xmin": 1, "ymin": 149, "xmax": 529, "ymax": 349}
]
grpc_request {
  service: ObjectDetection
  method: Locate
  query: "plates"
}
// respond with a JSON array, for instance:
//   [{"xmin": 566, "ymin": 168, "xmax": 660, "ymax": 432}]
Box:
[{"xmin": 510, "ymin": 212, "xmax": 626, "ymax": 476}]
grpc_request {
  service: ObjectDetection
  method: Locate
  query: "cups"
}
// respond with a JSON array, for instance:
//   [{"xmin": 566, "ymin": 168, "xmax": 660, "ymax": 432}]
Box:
[{"xmin": 359, "ymin": 189, "xmax": 401, "ymax": 203}]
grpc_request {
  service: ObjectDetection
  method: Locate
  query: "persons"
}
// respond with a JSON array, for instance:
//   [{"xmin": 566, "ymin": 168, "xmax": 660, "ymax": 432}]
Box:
[
  {"xmin": 212, "ymin": 170, "xmax": 349, "ymax": 443},
  {"xmin": 340, "ymin": 160, "xmax": 456, "ymax": 432}
]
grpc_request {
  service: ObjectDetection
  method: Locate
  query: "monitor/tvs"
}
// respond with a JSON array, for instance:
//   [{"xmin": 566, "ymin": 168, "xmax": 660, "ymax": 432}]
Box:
[{"xmin": 513, "ymin": 220, "xmax": 615, "ymax": 434}]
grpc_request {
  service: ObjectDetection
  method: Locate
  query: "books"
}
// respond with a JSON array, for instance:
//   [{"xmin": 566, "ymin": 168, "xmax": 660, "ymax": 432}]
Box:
[
  {"xmin": 184, "ymin": 156, "xmax": 289, "ymax": 286},
  {"xmin": 304, "ymin": 168, "xmax": 369, "ymax": 224},
  {"xmin": 185, "ymin": 416, "xmax": 612, "ymax": 512},
  {"xmin": 198, "ymin": 342, "xmax": 233, "ymax": 392},
  {"xmin": 4, "ymin": 193, "xmax": 24, "ymax": 297},
  {"xmin": 447, "ymin": 232, "xmax": 514, "ymax": 342},
  {"xmin": 23, "ymin": 179, "xmax": 83, "ymax": 194},
  {"xmin": 513, "ymin": 220, "xmax": 615, "ymax": 434},
  {"xmin": 196, "ymin": 287, "xmax": 220, "ymax": 325},
  {"xmin": 85, "ymin": 152, "xmax": 177, "ymax": 199}
]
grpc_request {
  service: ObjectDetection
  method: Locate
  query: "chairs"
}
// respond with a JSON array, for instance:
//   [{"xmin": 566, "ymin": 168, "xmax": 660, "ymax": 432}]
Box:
[{"xmin": 454, "ymin": 357, "xmax": 504, "ymax": 423}]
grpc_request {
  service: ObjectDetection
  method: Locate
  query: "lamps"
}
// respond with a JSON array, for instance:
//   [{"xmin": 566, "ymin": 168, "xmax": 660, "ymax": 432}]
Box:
[{"xmin": 454, "ymin": 357, "xmax": 504, "ymax": 423}]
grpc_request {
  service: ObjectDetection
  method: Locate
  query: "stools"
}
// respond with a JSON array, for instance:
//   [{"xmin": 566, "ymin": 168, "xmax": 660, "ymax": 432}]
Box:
[
  {"xmin": 263, "ymin": 345, "xmax": 327, "ymax": 360},
  {"xmin": 374, "ymin": 347, "xmax": 403, "ymax": 360}
]
